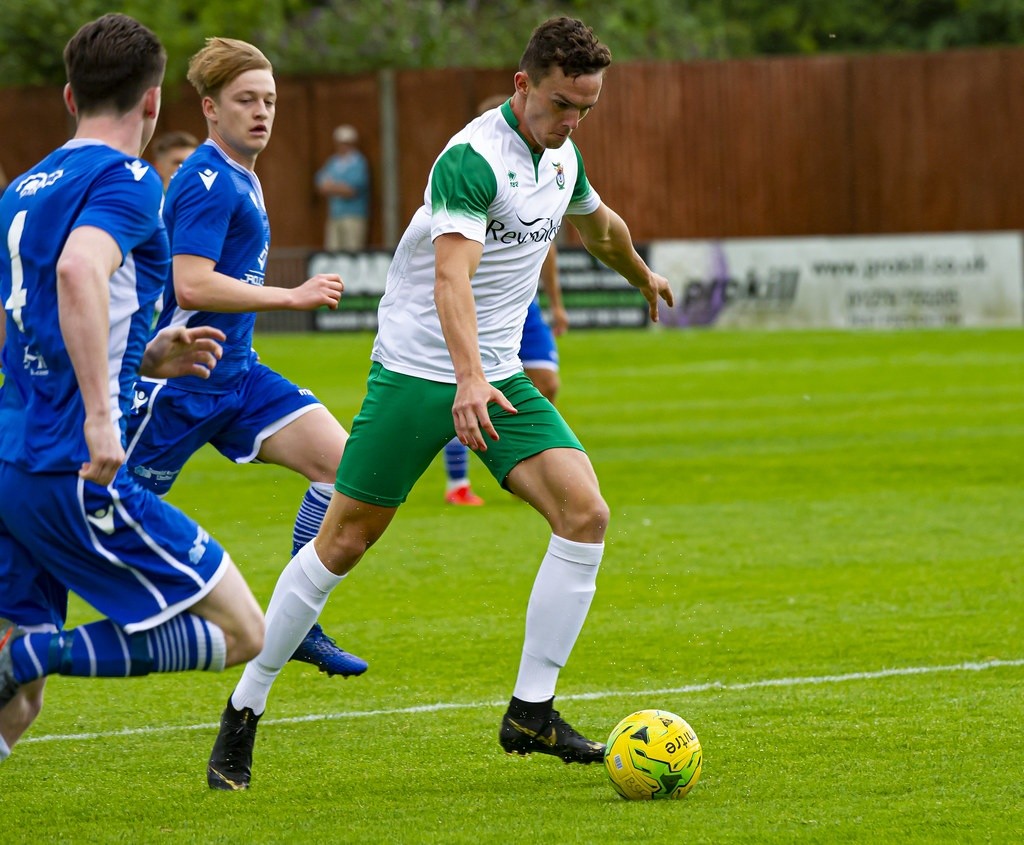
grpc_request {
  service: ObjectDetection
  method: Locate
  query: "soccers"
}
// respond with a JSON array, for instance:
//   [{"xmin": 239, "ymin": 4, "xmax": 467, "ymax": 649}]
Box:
[{"xmin": 604, "ymin": 708, "xmax": 705, "ymax": 802}]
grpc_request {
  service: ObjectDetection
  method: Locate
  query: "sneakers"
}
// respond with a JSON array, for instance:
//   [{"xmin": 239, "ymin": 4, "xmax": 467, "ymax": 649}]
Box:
[
  {"xmin": 446, "ymin": 478, "xmax": 482, "ymax": 505},
  {"xmin": 0, "ymin": 616, "xmax": 26, "ymax": 712},
  {"xmin": 499, "ymin": 709, "xmax": 606, "ymax": 766},
  {"xmin": 288, "ymin": 622, "xmax": 369, "ymax": 681},
  {"xmin": 207, "ymin": 709, "xmax": 264, "ymax": 793}
]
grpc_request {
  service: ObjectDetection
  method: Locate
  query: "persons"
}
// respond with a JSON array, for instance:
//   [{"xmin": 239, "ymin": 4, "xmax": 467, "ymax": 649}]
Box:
[
  {"xmin": 446, "ymin": 94, "xmax": 567, "ymax": 504},
  {"xmin": 205, "ymin": 16, "xmax": 672, "ymax": 799},
  {"xmin": 153, "ymin": 130, "xmax": 199, "ymax": 194},
  {"xmin": 122, "ymin": 32, "xmax": 368, "ymax": 680},
  {"xmin": 0, "ymin": 11, "xmax": 268, "ymax": 766},
  {"xmin": 314, "ymin": 124, "xmax": 370, "ymax": 254}
]
